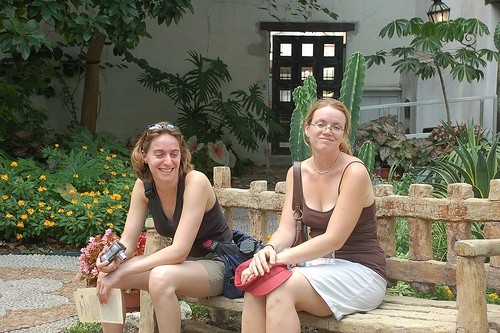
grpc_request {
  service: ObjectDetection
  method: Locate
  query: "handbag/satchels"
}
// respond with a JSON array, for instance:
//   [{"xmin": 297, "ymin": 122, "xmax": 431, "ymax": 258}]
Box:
[
  {"xmin": 217, "ymin": 240, "xmax": 243, "ymax": 299},
  {"xmin": 292, "ymin": 160, "xmax": 303, "ymax": 265}
]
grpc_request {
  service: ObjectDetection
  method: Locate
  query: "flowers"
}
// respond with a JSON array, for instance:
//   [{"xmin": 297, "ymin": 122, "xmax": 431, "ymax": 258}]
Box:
[{"xmin": 75, "ymin": 224, "xmax": 147, "ymax": 288}]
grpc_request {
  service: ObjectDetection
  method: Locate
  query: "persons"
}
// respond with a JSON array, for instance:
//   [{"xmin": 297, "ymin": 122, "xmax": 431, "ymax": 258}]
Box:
[
  {"xmin": 95, "ymin": 121, "xmax": 244, "ymax": 333},
  {"xmin": 241, "ymin": 98, "xmax": 387, "ymax": 333}
]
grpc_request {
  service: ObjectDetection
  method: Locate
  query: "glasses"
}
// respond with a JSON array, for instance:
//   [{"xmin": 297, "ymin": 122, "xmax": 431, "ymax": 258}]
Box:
[
  {"xmin": 141, "ymin": 124, "xmax": 178, "ymax": 151},
  {"xmin": 309, "ymin": 123, "xmax": 345, "ymax": 135}
]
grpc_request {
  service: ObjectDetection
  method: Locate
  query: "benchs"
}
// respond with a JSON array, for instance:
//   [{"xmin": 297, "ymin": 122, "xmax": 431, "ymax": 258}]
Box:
[{"xmin": 138, "ymin": 157, "xmax": 500, "ymax": 333}]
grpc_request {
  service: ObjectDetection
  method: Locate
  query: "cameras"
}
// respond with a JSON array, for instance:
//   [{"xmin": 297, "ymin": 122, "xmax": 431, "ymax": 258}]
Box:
[{"xmin": 101, "ymin": 241, "xmax": 128, "ymax": 265}]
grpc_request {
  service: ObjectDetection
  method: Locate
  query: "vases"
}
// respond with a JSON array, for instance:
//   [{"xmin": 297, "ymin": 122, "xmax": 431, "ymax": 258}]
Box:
[{"xmin": 71, "ymin": 286, "xmax": 141, "ymax": 333}]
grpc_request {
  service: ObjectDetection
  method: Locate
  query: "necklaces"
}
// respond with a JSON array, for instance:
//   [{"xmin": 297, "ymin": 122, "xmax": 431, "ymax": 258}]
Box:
[{"xmin": 310, "ymin": 151, "xmax": 341, "ymax": 174}]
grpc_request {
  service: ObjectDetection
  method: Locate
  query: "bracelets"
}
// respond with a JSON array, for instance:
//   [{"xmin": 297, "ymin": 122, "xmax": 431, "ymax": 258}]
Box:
[{"xmin": 264, "ymin": 244, "xmax": 274, "ymax": 250}]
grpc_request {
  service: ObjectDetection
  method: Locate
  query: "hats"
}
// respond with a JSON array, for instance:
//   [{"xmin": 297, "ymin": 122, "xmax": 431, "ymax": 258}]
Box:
[{"xmin": 233, "ymin": 257, "xmax": 292, "ymax": 295}]
